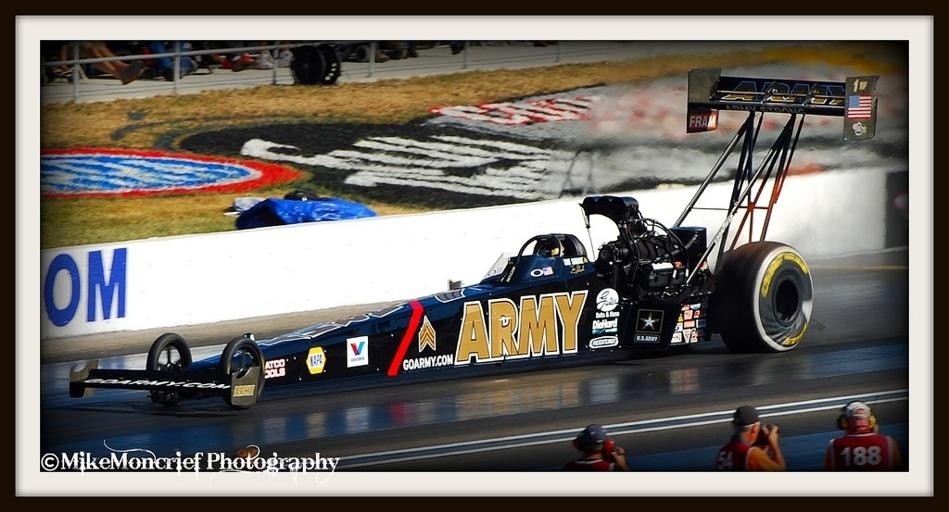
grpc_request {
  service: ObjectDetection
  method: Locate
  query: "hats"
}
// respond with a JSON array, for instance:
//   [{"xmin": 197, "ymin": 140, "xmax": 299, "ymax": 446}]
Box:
[
  {"xmin": 577, "ymin": 424, "xmax": 606, "ymax": 443},
  {"xmin": 734, "ymin": 406, "xmax": 759, "ymax": 425},
  {"xmin": 845, "ymin": 402, "xmax": 871, "ymax": 419}
]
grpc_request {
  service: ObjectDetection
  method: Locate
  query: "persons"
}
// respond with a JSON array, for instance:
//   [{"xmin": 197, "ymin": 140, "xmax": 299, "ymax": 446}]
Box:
[
  {"xmin": 562, "ymin": 424, "xmax": 631, "ymax": 471},
  {"xmin": 550, "ymin": 237, "xmax": 560, "ymax": 257},
  {"xmin": 823, "ymin": 400, "xmax": 901, "ymax": 472},
  {"xmin": 40, "ymin": 41, "xmax": 390, "ymax": 84},
  {"xmin": 712, "ymin": 406, "xmax": 789, "ymax": 471}
]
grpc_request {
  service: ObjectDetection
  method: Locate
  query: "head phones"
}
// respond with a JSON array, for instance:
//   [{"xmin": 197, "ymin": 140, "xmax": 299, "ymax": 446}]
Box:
[
  {"xmin": 836, "ymin": 399, "xmax": 877, "ymax": 429},
  {"xmin": 573, "ymin": 423, "xmax": 614, "ymax": 455}
]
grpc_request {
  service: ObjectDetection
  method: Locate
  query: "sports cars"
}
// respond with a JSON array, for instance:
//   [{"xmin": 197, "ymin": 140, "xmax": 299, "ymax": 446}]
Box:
[{"xmin": 69, "ymin": 68, "xmax": 880, "ymax": 410}]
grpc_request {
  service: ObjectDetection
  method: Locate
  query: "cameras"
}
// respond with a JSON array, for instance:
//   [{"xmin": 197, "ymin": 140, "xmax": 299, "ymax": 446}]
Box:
[{"xmin": 760, "ymin": 423, "xmax": 779, "ymax": 442}]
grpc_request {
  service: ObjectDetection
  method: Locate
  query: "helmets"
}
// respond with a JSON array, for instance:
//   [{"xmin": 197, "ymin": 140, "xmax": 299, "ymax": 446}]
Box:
[{"xmin": 537, "ymin": 239, "xmax": 567, "ymax": 258}]
